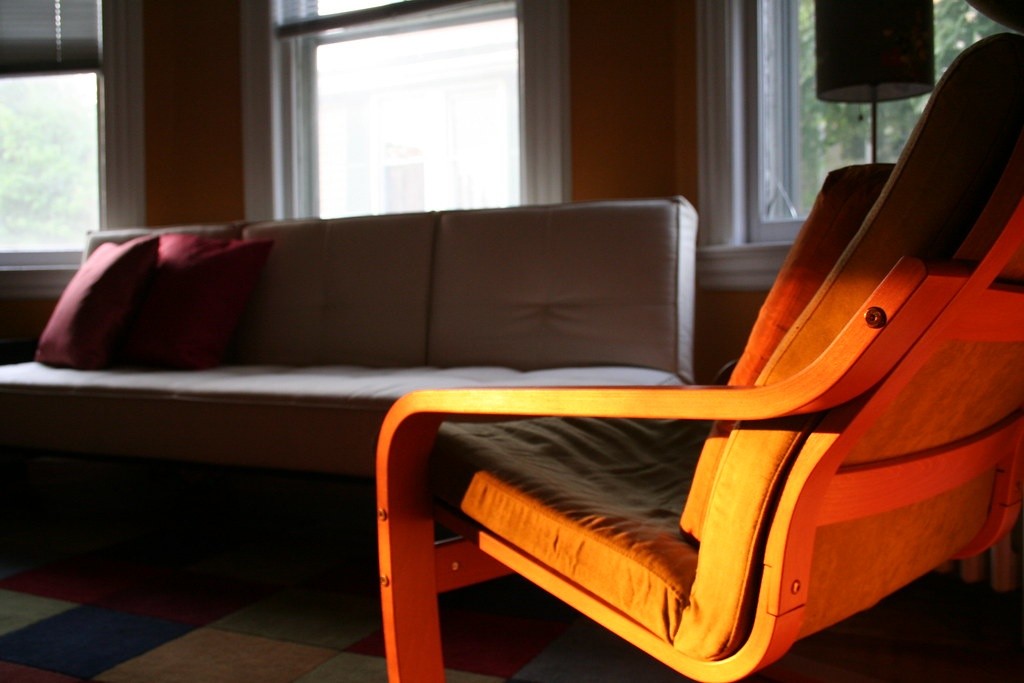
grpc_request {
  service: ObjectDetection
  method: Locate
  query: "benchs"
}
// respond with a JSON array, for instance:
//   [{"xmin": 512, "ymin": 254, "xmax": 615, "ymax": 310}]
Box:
[{"xmin": 0, "ymin": 194, "xmax": 699, "ymax": 481}]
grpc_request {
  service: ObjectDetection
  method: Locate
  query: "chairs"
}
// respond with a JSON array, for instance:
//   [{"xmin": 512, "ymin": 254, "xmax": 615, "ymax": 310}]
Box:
[{"xmin": 376, "ymin": 0, "xmax": 1024, "ymax": 683}]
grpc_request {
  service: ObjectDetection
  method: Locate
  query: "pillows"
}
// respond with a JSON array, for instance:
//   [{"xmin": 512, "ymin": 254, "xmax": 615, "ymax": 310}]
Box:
[
  {"xmin": 122, "ymin": 234, "xmax": 276, "ymax": 371},
  {"xmin": 36, "ymin": 234, "xmax": 160, "ymax": 370}
]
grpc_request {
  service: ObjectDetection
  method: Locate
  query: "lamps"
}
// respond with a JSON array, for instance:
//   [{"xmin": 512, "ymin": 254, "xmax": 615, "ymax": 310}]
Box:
[{"xmin": 814, "ymin": 0, "xmax": 935, "ymax": 165}]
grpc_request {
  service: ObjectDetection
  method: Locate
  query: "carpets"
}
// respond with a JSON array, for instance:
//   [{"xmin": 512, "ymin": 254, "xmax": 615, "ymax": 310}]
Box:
[{"xmin": 0, "ymin": 540, "xmax": 816, "ymax": 683}]
